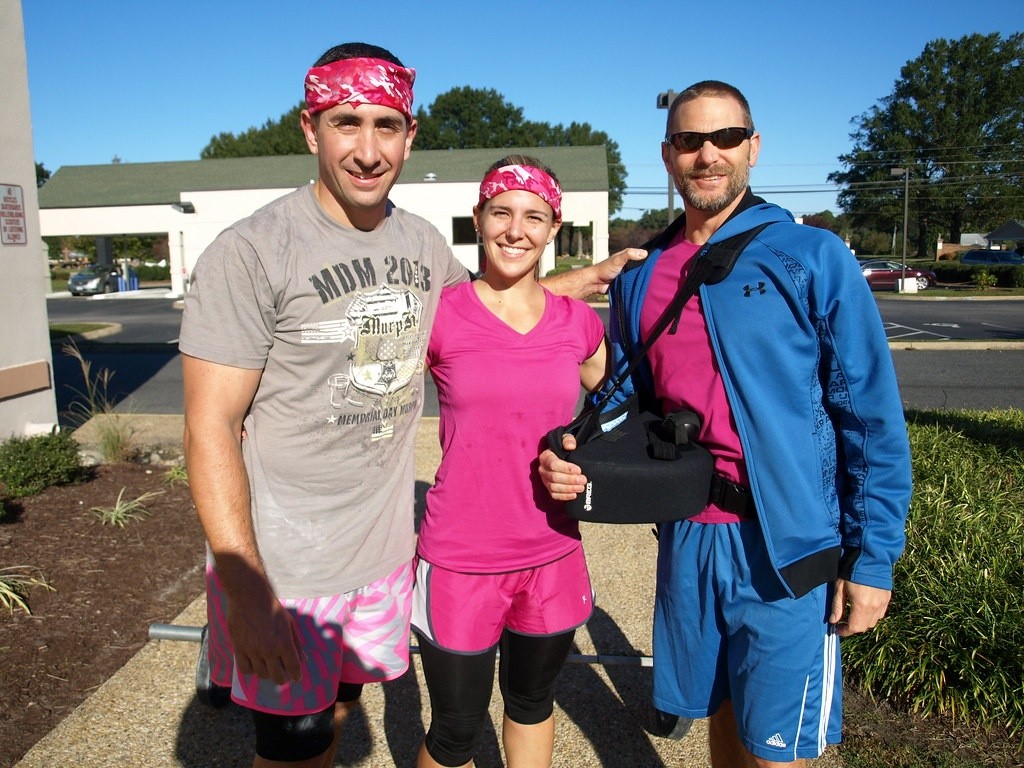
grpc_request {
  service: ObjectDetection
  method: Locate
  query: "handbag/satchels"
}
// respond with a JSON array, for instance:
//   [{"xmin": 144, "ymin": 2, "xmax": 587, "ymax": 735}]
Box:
[{"xmin": 547, "ymin": 389, "xmax": 714, "ymax": 524}]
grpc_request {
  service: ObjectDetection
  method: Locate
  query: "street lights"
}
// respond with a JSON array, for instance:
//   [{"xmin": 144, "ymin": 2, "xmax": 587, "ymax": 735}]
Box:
[
  {"xmin": 657, "ymin": 88, "xmax": 682, "ymax": 229},
  {"xmin": 889, "ymin": 167, "xmax": 910, "ymax": 293}
]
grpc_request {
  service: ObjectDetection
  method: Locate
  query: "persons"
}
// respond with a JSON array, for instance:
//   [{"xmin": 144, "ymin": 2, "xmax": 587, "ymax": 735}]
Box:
[
  {"xmin": 416, "ymin": 152, "xmax": 605, "ymax": 768},
  {"xmin": 535, "ymin": 82, "xmax": 916, "ymax": 767},
  {"xmin": 178, "ymin": 43, "xmax": 650, "ymax": 768}
]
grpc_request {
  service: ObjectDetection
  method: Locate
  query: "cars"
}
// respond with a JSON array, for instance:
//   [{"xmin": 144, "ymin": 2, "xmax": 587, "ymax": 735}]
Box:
[
  {"xmin": 959, "ymin": 248, "xmax": 1024, "ymax": 266},
  {"xmin": 860, "ymin": 260, "xmax": 937, "ymax": 291},
  {"xmin": 68, "ymin": 263, "xmax": 119, "ymax": 298}
]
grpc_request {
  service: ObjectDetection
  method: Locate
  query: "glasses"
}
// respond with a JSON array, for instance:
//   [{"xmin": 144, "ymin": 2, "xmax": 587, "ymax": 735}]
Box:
[{"xmin": 664, "ymin": 127, "xmax": 753, "ymax": 153}]
